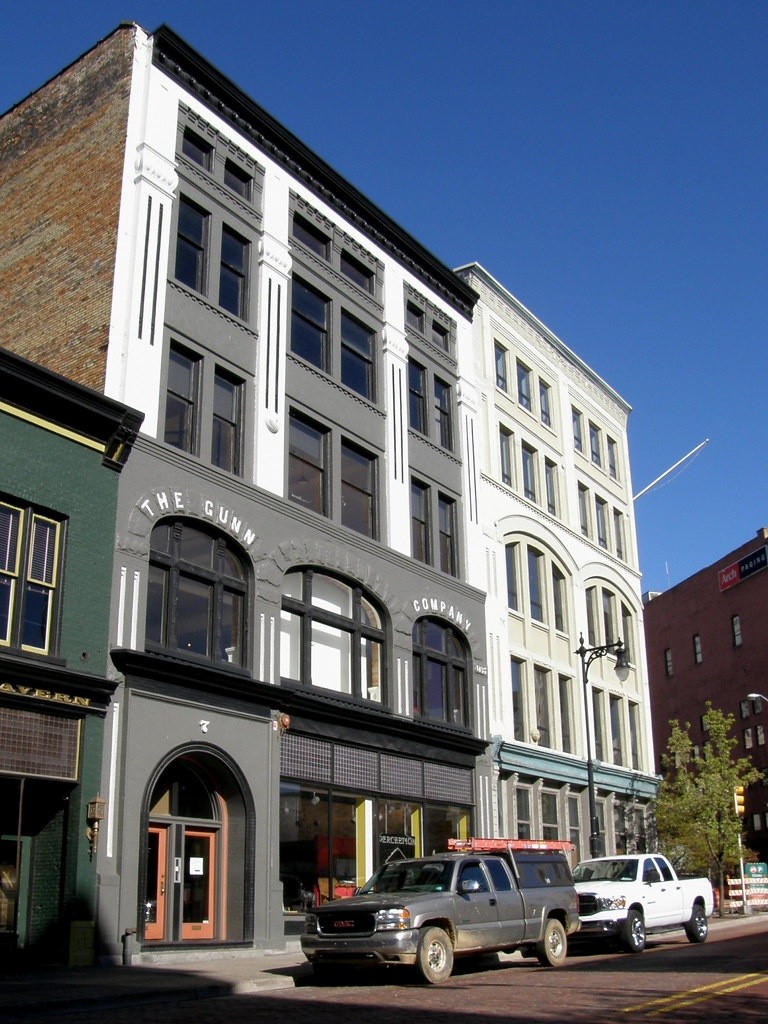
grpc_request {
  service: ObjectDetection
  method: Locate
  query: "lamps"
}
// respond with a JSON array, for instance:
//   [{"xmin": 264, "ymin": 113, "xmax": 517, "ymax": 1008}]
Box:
[{"xmin": 85, "ymin": 792, "xmax": 107, "ymax": 854}]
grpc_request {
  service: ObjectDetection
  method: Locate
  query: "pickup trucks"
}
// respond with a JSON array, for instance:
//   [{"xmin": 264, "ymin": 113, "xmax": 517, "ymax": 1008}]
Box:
[{"xmin": 563, "ymin": 854, "xmax": 715, "ymax": 952}]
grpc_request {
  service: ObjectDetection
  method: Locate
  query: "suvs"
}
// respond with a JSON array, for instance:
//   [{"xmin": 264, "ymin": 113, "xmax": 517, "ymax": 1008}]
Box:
[{"xmin": 300, "ymin": 837, "xmax": 582, "ymax": 985}]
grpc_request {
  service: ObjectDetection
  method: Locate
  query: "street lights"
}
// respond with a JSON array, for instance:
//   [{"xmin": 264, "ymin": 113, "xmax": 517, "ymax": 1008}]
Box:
[{"xmin": 572, "ymin": 632, "xmax": 632, "ymax": 857}]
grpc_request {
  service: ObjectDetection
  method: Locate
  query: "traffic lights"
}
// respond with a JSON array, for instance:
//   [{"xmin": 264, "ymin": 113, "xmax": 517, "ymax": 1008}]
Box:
[{"xmin": 732, "ymin": 785, "xmax": 746, "ymax": 817}]
